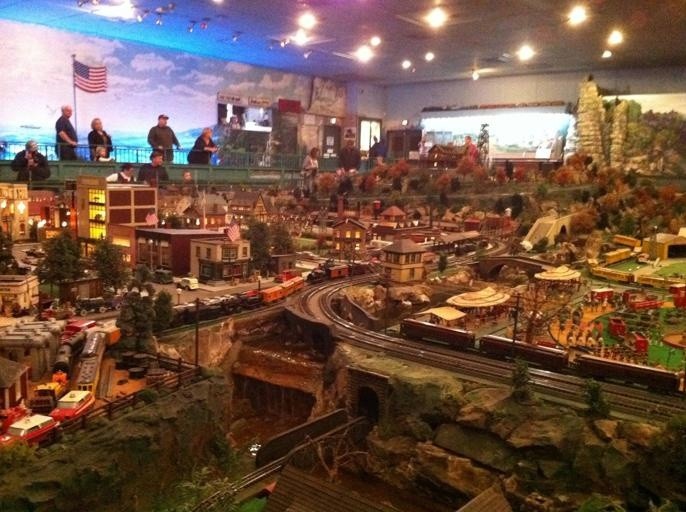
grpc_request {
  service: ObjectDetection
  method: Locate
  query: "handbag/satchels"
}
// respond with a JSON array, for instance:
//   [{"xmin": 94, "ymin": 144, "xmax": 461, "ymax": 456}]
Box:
[{"xmin": 300, "ymin": 170, "xmax": 313, "ymax": 178}]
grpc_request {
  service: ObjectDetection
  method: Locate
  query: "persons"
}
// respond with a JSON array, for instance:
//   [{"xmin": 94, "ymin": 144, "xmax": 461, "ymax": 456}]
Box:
[
  {"xmin": 11, "ymin": 141, "xmax": 51, "ymax": 181},
  {"xmin": 55, "ymin": 105, "xmax": 78, "ymax": 160},
  {"xmin": 182, "ymin": 170, "xmax": 193, "ymax": 184},
  {"xmin": 338, "ymin": 141, "xmax": 360, "ymax": 172},
  {"xmin": 138, "ymin": 152, "xmax": 170, "ymax": 185},
  {"xmin": 369, "ymin": 135, "xmax": 386, "ymax": 166},
  {"xmin": 88, "ymin": 119, "xmax": 113, "ymax": 162},
  {"xmin": 187, "ymin": 127, "xmax": 218, "ymax": 164},
  {"xmin": 94, "ymin": 147, "xmax": 113, "ymax": 161},
  {"xmin": 148, "ymin": 115, "xmax": 182, "ymax": 161},
  {"xmin": 416, "ymin": 138, "xmax": 430, "ymax": 169},
  {"xmin": 464, "ymin": 136, "xmax": 479, "ymax": 161},
  {"xmin": 301, "ymin": 148, "xmax": 319, "ymax": 180},
  {"xmin": 106, "ymin": 163, "xmax": 138, "ymax": 184}
]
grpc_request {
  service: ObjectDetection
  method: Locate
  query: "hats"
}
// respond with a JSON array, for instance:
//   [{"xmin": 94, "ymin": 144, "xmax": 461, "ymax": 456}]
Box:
[
  {"xmin": 159, "ymin": 115, "xmax": 168, "ymax": 119},
  {"xmin": 26, "ymin": 140, "xmax": 40, "ymax": 153}
]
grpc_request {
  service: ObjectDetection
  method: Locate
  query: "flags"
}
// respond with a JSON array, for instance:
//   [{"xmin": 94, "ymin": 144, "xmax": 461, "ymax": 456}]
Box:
[
  {"xmin": 71, "ymin": 54, "xmax": 106, "ymax": 92},
  {"xmin": 227, "ymin": 216, "xmax": 241, "ymax": 242},
  {"xmin": 145, "ymin": 213, "xmax": 159, "ymax": 227}
]
